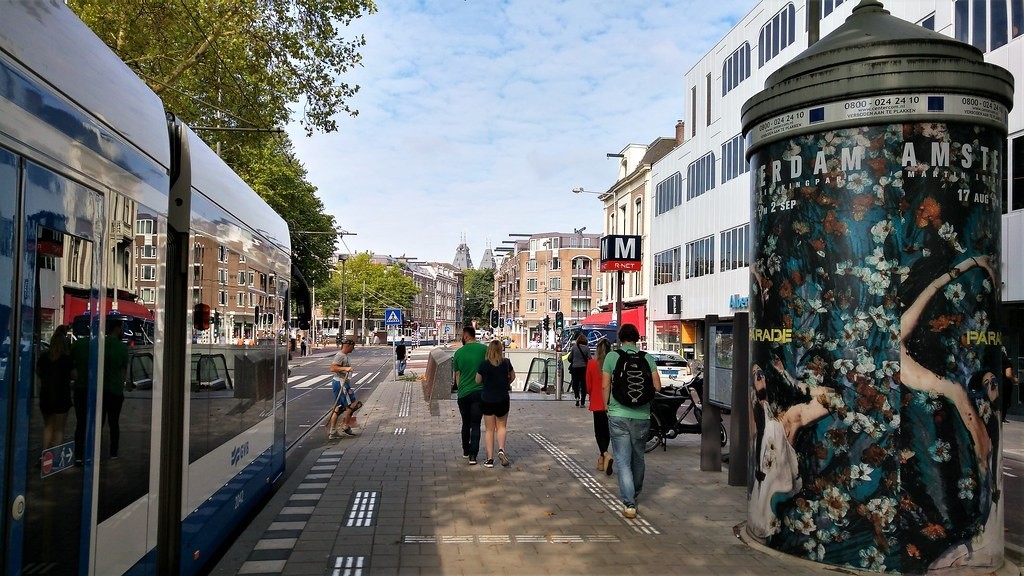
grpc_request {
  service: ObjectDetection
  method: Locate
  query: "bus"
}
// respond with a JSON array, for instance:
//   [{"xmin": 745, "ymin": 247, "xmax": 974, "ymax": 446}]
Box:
[{"xmin": 0, "ymin": 0, "xmax": 315, "ymax": 576}]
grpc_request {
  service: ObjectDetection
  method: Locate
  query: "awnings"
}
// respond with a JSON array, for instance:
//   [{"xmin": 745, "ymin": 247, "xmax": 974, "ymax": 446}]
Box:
[
  {"xmin": 582, "ymin": 309, "xmax": 639, "ymax": 332},
  {"xmin": 104, "ymin": 297, "xmax": 152, "ymax": 321}
]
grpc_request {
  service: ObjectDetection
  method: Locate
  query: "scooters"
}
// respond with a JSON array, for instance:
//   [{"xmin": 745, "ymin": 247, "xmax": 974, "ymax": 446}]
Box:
[{"xmin": 643, "ymin": 371, "xmax": 727, "ymax": 453}]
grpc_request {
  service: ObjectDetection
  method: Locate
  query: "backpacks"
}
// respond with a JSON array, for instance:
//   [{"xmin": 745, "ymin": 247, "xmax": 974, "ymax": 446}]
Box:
[
  {"xmin": 301, "ymin": 342, "xmax": 304, "ymax": 346},
  {"xmin": 612, "ymin": 349, "xmax": 655, "ymax": 408}
]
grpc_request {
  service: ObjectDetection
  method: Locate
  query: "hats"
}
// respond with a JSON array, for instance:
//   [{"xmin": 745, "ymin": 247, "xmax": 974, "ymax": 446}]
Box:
[{"xmin": 343, "ymin": 339, "xmax": 356, "ymax": 346}]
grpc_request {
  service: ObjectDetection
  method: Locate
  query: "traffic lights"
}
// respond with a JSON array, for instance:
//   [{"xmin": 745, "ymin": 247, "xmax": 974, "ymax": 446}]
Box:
[
  {"xmin": 555, "ymin": 311, "xmax": 563, "ymax": 329},
  {"xmin": 209, "ymin": 317, "xmax": 213, "ymax": 324},
  {"xmin": 543, "ymin": 318, "xmax": 547, "ymax": 330},
  {"xmin": 263, "ymin": 313, "xmax": 267, "ymax": 324},
  {"xmin": 254, "ymin": 304, "xmax": 260, "ymax": 325},
  {"xmin": 499, "ymin": 318, "xmax": 504, "ymax": 328},
  {"xmin": 547, "ymin": 315, "xmax": 552, "ymax": 335},
  {"xmin": 214, "ymin": 311, "xmax": 220, "ymax": 326},
  {"xmin": 244, "ymin": 321, "xmax": 247, "ymax": 327},
  {"xmin": 268, "ymin": 313, "xmax": 274, "ymax": 324},
  {"xmin": 491, "ymin": 309, "xmax": 499, "ymax": 328}
]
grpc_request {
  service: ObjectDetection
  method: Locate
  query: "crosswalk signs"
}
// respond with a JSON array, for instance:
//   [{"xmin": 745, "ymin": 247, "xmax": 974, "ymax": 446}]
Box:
[{"xmin": 385, "ymin": 308, "xmax": 402, "ymax": 325}]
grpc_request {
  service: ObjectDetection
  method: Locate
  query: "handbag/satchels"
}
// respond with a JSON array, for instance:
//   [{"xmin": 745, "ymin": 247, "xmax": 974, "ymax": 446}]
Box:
[
  {"xmin": 584, "ymin": 356, "xmax": 589, "ymax": 363},
  {"xmin": 395, "ymin": 361, "xmax": 398, "ymax": 369}
]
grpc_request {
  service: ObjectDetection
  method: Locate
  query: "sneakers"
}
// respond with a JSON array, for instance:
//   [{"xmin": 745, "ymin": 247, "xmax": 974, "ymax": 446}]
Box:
[
  {"xmin": 596, "ymin": 460, "xmax": 605, "ymax": 471},
  {"xmin": 483, "ymin": 459, "xmax": 494, "ymax": 468},
  {"xmin": 576, "ymin": 397, "xmax": 579, "ymax": 406},
  {"xmin": 328, "ymin": 431, "xmax": 343, "ymax": 439},
  {"xmin": 625, "ymin": 507, "xmax": 636, "ymax": 518},
  {"xmin": 342, "ymin": 428, "xmax": 355, "ymax": 437},
  {"xmin": 468, "ymin": 457, "xmax": 477, "ymax": 464},
  {"xmin": 463, "ymin": 453, "xmax": 469, "ymax": 458},
  {"xmin": 580, "ymin": 405, "xmax": 585, "ymax": 408},
  {"xmin": 498, "ymin": 449, "xmax": 509, "ymax": 466},
  {"xmin": 604, "ymin": 454, "xmax": 614, "ymax": 476}
]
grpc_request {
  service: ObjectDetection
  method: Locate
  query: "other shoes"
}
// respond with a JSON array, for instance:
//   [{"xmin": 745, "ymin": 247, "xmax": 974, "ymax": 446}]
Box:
[
  {"xmin": 111, "ymin": 456, "xmax": 118, "ymax": 460},
  {"xmin": 398, "ymin": 372, "xmax": 404, "ymax": 376},
  {"xmin": 76, "ymin": 460, "xmax": 82, "ymax": 462}
]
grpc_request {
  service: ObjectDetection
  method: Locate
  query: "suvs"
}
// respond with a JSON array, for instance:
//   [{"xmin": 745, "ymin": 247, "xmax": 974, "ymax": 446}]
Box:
[{"xmin": 551, "ymin": 322, "xmax": 620, "ymax": 354}]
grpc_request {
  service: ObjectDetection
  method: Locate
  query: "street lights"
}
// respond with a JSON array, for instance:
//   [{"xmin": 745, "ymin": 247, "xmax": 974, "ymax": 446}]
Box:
[
  {"xmin": 572, "ymin": 187, "xmax": 616, "ymax": 320},
  {"xmin": 337, "ymin": 255, "xmax": 348, "ymax": 346}
]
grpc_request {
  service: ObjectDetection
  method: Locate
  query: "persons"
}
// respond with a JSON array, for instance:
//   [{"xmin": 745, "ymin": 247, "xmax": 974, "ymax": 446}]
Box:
[
  {"xmin": 300, "ymin": 337, "xmax": 313, "ymax": 356},
  {"xmin": 35, "ymin": 315, "xmax": 129, "ymax": 466},
  {"xmin": 475, "ymin": 340, "xmax": 515, "ymax": 469},
  {"xmin": 395, "ymin": 338, "xmax": 408, "ymax": 376},
  {"xmin": 328, "ymin": 339, "xmax": 358, "ymax": 440},
  {"xmin": 586, "ymin": 338, "xmax": 614, "ymax": 475},
  {"xmin": 751, "ymin": 255, "xmax": 1004, "ymax": 574},
  {"xmin": 452, "ymin": 326, "xmax": 489, "ymax": 464},
  {"xmin": 600, "ymin": 323, "xmax": 662, "ymax": 519},
  {"xmin": 484, "ymin": 333, "xmax": 516, "ymax": 349},
  {"xmin": 568, "ymin": 334, "xmax": 592, "ymax": 408}
]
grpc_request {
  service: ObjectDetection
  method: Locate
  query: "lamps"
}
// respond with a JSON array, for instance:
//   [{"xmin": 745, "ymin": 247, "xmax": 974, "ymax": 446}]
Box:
[{"xmin": 573, "ymin": 187, "xmax": 616, "ymax": 194}]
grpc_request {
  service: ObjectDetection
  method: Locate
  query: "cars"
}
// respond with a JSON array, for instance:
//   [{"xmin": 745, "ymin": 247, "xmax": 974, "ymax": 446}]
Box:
[
  {"xmin": 642, "ymin": 350, "xmax": 692, "ymax": 387},
  {"xmin": 190, "ymin": 352, "xmax": 232, "ymax": 391},
  {"xmin": 125, "ymin": 352, "xmax": 155, "ymax": 391}
]
